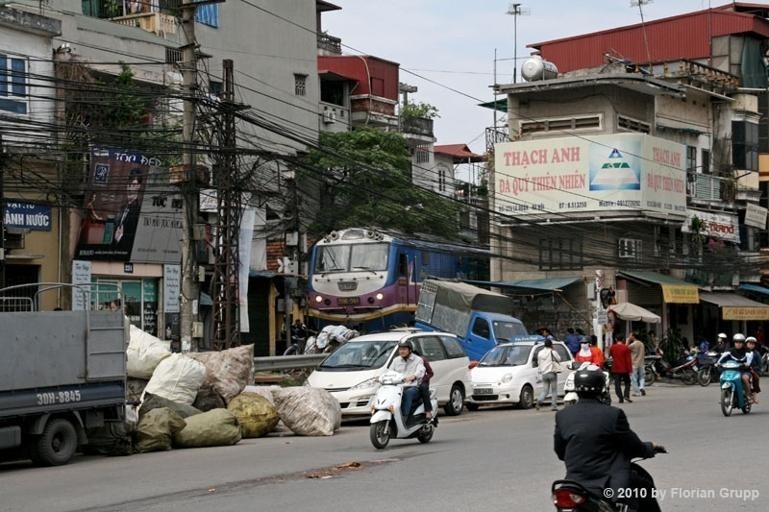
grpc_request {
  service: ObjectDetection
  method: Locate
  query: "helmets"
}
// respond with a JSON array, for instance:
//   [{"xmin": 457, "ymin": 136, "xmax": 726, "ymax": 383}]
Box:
[
  {"xmin": 574, "ymin": 365, "xmax": 606, "ymax": 396},
  {"xmin": 398, "ymin": 341, "xmax": 412, "ymax": 349},
  {"xmin": 718, "ymin": 333, "xmax": 757, "ymax": 343}
]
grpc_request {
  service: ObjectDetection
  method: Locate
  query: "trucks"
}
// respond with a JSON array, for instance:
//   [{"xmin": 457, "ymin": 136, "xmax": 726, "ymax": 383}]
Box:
[
  {"xmin": 0, "ymin": 276, "xmax": 131, "ymax": 474},
  {"xmin": 409, "ymin": 274, "xmax": 528, "ymax": 366}
]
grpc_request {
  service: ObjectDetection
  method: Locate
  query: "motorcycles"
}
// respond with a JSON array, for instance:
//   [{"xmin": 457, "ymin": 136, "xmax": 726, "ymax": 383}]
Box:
[
  {"xmin": 361, "ymin": 365, "xmax": 440, "ymax": 447},
  {"xmin": 758, "ymin": 341, "xmax": 769, "ymax": 375},
  {"xmin": 637, "ymin": 336, "xmax": 728, "ymax": 386},
  {"xmin": 549, "ymin": 447, "xmax": 666, "ymax": 512},
  {"xmin": 562, "ymin": 360, "xmax": 611, "ymax": 409},
  {"xmin": 709, "ymin": 360, "xmax": 755, "ymax": 415}
]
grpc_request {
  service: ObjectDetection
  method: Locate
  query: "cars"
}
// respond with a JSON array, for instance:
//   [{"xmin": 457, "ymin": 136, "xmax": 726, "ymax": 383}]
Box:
[
  {"xmin": 464, "ymin": 338, "xmax": 577, "ymax": 412},
  {"xmin": 305, "ymin": 322, "xmax": 474, "ymax": 421}
]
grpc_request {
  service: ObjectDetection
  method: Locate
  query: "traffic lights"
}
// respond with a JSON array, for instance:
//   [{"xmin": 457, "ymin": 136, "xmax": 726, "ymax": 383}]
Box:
[{"xmin": 598, "ymin": 286, "xmax": 616, "ymax": 309}]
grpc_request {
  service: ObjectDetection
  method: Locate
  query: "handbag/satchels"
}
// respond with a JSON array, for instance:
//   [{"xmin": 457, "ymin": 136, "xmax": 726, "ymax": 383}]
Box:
[{"xmin": 552, "ymin": 360, "xmax": 561, "ymax": 373}]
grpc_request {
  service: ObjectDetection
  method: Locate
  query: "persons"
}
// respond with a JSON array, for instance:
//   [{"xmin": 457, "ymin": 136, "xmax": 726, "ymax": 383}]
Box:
[
  {"xmin": 111, "ymin": 168, "xmax": 143, "ymax": 250},
  {"xmin": 291, "ymin": 319, "xmax": 308, "ymax": 355},
  {"xmin": 553, "ymin": 364, "xmax": 661, "ymax": 511},
  {"xmin": 82, "ymin": 191, "xmax": 104, "ymax": 230},
  {"xmin": 111, "ymin": 299, "xmax": 128, "ymax": 321},
  {"xmin": 536, "ymin": 326, "xmax": 762, "ymax": 412},
  {"xmin": 411, "ymin": 341, "xmax": 434, "ymax": 418},
  {"xmin": 374, "ymin": 340, "xmax": 426, "ymax": 422}
]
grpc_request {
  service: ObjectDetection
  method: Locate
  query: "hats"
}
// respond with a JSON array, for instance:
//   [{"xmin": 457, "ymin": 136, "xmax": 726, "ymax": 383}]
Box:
[{"xmin": 579, "ymin": 337, "xmax": 592, "ymax": 343}]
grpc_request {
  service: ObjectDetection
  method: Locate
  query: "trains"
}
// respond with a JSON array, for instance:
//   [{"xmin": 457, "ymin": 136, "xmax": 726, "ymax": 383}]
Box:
[{"xmin": 309, "ymin": 224, "xmax": 492, "ymax": 336}]
